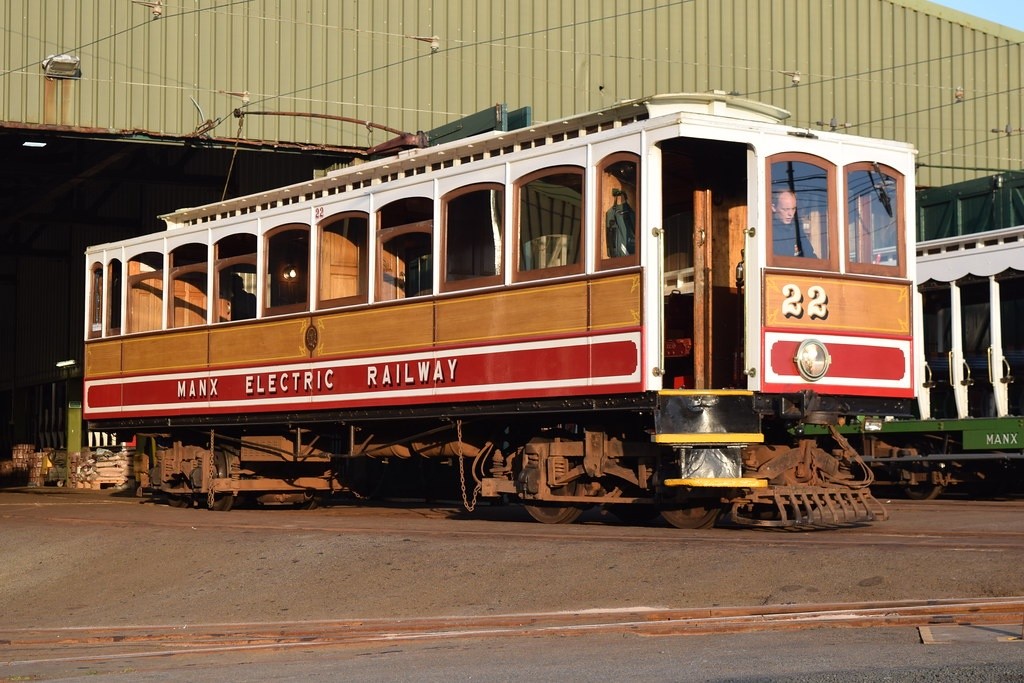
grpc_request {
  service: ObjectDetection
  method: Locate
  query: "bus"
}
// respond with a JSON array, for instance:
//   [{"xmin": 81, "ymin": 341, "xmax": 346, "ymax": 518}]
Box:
[
  {"xmin": 779, "ymin": 223, "xmax": 1023, "ymax": 492},
  {"xmin": 82, "ymin": 95, "xmax": 923, "ymax": 526}
]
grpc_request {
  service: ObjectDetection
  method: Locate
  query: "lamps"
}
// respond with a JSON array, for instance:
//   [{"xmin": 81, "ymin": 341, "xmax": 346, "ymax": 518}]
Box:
[
  {"xmin": 42, "ymin": 53, "xmax": 81, "ymax": 80},
  {"xmin": 284, "ymin": 264, "xmax": 297, "ymax": 279}
]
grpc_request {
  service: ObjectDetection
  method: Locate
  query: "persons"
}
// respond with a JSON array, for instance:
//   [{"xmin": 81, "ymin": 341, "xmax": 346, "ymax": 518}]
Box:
[
  {"xmin": 773, "ymin": 190, "xmax": 818, "ymax": 258},
  {"xmin": 232, "ymin": 274, "xmax": 256, "ymax": 320}
]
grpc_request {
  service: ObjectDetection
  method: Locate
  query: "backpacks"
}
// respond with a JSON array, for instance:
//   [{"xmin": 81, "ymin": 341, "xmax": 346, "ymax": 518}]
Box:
[{"xmin": 606, "ymin": 191, "xmax": 636, "ymax": 259}]
